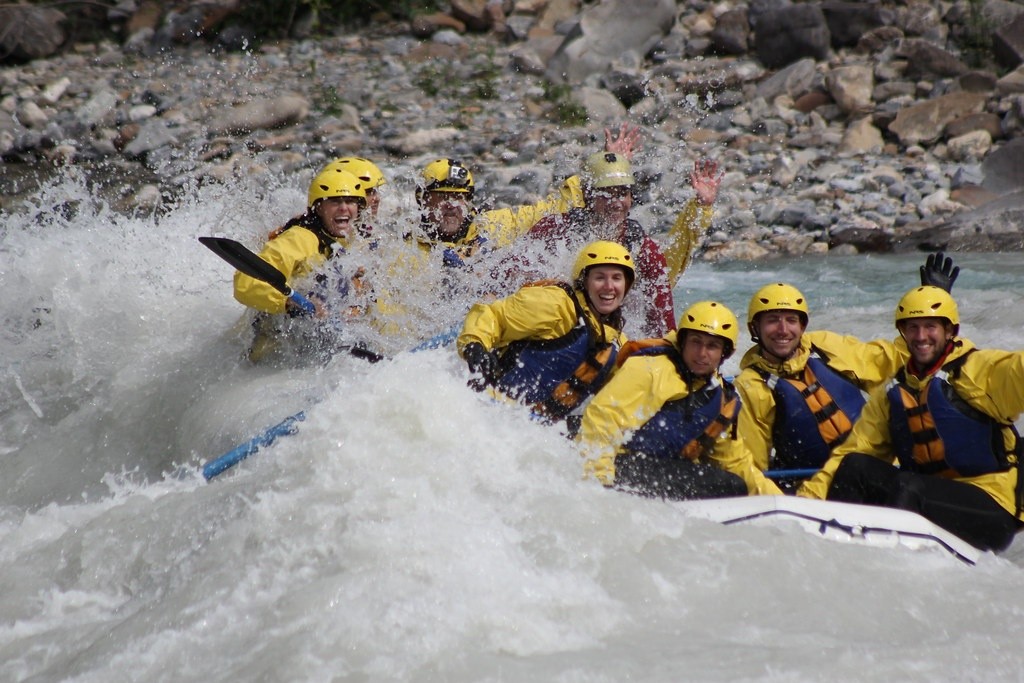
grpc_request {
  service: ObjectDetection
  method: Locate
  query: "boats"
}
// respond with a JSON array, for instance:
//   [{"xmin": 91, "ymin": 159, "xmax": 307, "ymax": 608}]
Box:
[{"xmin": 177, "ymin": 361, "xmax": 986, "ymax": 567}]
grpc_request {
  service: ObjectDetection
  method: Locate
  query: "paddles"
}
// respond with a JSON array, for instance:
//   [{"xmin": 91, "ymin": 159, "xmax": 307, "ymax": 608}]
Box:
[
  {"xmin": 764, "ymin": 469, "xmax": 822, "ymax": 478},
  {"xmin": 198, "ymin": 236, "xmax": 322, "ymax": 316},
  {"xmin": 198, "ymin": 324, "xmax": 464, "ymax": 478}
]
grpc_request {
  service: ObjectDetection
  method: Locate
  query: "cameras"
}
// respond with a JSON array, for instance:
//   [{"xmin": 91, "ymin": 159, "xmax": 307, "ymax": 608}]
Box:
[{"xmin": 448, "ymin": 166, "xmax": 468, "ymax": 182}]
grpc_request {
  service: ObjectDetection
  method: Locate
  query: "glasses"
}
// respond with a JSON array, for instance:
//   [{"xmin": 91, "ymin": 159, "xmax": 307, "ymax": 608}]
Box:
[{"xmin": 597, "ymin": 187, "xmax": 629, "ymax": 199}]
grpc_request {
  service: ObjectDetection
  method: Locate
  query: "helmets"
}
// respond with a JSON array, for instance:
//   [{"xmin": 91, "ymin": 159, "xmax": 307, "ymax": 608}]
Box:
[
  {"xmin": 676, "ymin": 301, "xmax": 738, "ymax": 358},
  {"xmin": 316, "ymin": 156, "xmax": 387, "ymax": 191},
  {"xmin": 307, "ymin": 169, "xmax": 367, "ymax": 214},
  {"xmin": 893, "ymin": 285, "xmax": 960, "ymax": 341},
  {"xmin": 414, "ymin": 157, "xmax": 476, "ymax": 204},
  {"xmin": 580, "ymin": 150, "xmax": 637, "ymax": 190},
  {"xmin": 747, "ymin": 283, "xmax": 809, "ymax": 337},
  {"xmin": 571, "ymin": 240, "xmax": 636, "ymax": 300}
]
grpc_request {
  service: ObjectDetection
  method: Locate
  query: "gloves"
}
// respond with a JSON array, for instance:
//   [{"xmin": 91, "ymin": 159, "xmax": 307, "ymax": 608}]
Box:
[
  {"xmin": 920, "ymin": 253, "xmax": 961, "ymax": 294},
  {"xmin": 463, "ymin": 341, "xmax": 484, "ymax": 373}
]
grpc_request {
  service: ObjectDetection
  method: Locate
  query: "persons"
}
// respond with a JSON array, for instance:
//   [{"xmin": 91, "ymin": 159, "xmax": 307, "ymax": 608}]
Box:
[
  {"xmin": 234, "ymin": 157, "xmax": 387, "ymax": 362},
  {"xmin": 374, "ymin": 122, "xmax": 725, "ymax": 425},
  {"xmin": 797, "ymin": 284, "xmax": 1024, "ymax": 554},
  {"xmin": 575, "ymin": 301, "xmax": 784, "ymax": 499},
  {"xmin": 732, "ymin": 251, "xmax": 960, "ymax": 496}
]
